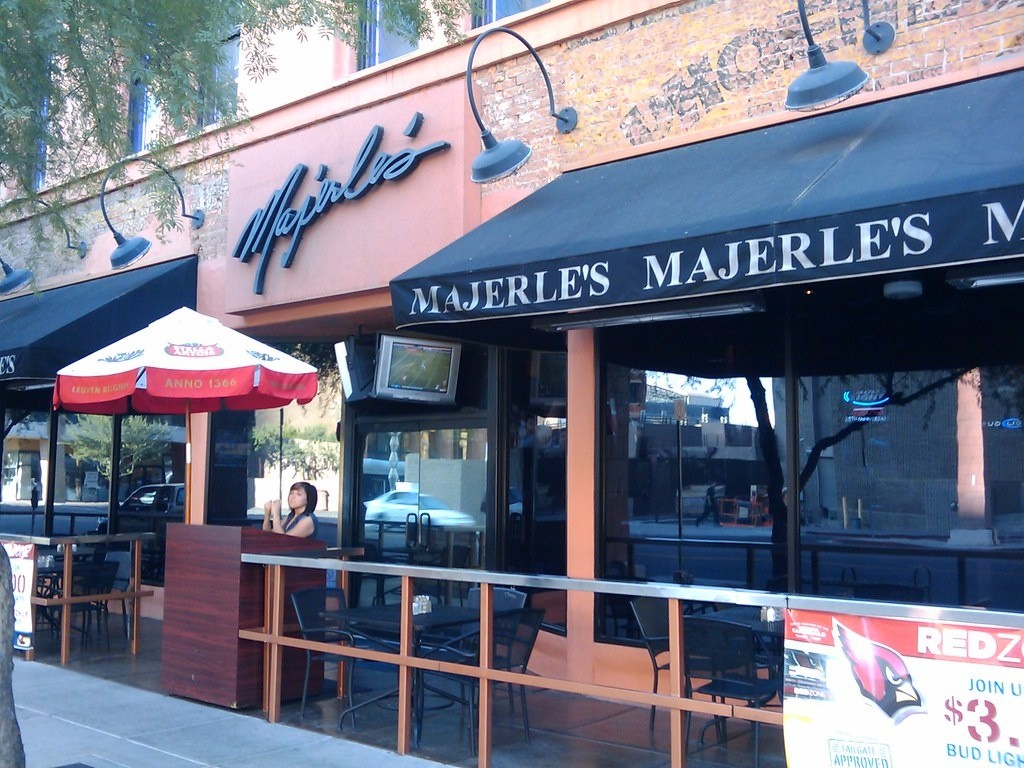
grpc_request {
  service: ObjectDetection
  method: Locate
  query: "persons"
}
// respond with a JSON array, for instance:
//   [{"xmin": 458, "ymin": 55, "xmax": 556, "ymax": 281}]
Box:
[
  {"xmin": 694, "ymin": 481, "xmax": 723, "ymax": 527},
  {"xmin": 262, "ymin": 482, "xmax": 318, "ymax": 539},
  {"xmin": 772, "ymin": 486, "xmax": 788, "ymax": 572}
]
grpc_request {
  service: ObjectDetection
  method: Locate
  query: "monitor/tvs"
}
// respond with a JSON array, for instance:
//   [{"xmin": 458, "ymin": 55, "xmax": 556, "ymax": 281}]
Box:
[
  {"xmin": 333, "ymin": 341, "xmax": 373, "ymax": 402},
  {"xmin": 530, "ymin": 350, "xmax": 569, "ymax": 406},
  {"xmin": 375, "ymin": 335, "xmax": 462, "ymax": 404}
]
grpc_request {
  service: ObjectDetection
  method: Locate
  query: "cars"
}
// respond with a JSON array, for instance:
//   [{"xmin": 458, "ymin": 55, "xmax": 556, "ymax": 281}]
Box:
[{"xmin": 363, "ymin": 490, "xmax": 478, "ymax": 551}]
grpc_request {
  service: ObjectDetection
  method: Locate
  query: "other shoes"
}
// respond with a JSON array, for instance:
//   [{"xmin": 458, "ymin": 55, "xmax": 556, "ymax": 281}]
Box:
[
  {"xmin": 714, "ymin": 524, "xmax": 723, "ymax": 527},
  {"xmin": 696, "ymin": 519, "xmax": 699, "ymax": 527}
]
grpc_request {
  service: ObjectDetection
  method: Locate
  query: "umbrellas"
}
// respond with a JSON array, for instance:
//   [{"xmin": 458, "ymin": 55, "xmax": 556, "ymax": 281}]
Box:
[{"xmin": 53, "ymin": 305, "xmax": 317, "ymax": 525}]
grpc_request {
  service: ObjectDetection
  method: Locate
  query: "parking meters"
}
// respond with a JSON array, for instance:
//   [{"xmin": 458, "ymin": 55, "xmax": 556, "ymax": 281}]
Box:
[{"xmin": 30, "ymin": 485, "xmax": 40, "ymax": 536}]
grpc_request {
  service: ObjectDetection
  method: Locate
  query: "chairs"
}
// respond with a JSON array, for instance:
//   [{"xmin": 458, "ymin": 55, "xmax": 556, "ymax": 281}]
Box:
[
  {"xmin": 606, "ymin": 557, "xmax": 658, "ymax": 638},
  {"xmin": 414, "ymin": 587, "xmax": 545, "ymax": 758},
  {"xmin": 629, "ymin": 596, "xmax": 786, "ymax": 767},
  {"xmin": 36, "ymin": 551, "xmax": 130, "ymax": 649},
  {"xmin": 438, "ymin": 545, "xmax": 470, "ymax": 607},
  {"xmin": 291, "ymin": 585, "xmax": 372, "ymax": 728}
]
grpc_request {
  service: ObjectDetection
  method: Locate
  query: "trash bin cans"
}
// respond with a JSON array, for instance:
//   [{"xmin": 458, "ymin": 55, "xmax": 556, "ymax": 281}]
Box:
[{"xmin": 676, "ymin": 492, "xmax": 708, "ymax": 515}]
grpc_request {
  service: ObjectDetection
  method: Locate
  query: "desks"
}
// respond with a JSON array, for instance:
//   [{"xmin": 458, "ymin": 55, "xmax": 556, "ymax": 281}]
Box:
[
  {"xmin": 696, "ymin": 605, "xmax": 784, "ymax": 748},
  {"xmin": 36, "ymin": 562, "xmax": 93, "ymax": 644},
  {"xmin": 35, "ymin": 547, "xmax": 98, "ymax": 560},
  {"xmin": 320, "ymin": 601, "xmax": 501, "ymax": 730}
]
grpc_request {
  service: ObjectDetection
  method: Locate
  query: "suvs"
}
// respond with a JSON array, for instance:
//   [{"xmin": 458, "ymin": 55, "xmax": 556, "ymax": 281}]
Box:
[{"xmin": 94, "ymin": 480, "xmax": 185, "ymax": 582}]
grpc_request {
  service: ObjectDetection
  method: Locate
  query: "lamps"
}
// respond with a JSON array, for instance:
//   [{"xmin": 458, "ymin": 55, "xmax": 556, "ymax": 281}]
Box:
[
  {"xmin": 781, "ymin": 0, "xmax": 897, "ymax": 108},
  {"xmin": 945, "ymin": 265, "xmax": 1024, "ymax": 289},
  {"xmin": 530, "ymin": 292, "xmax": 767, "ymax": 334},
  {"xmin": 466, "ymin": 27, "xmax": 578, "ymax": 182},
  {"xmin": 100, "ymin": 156, "xmax": 204, "ymax": 270},
  {"xmin": 0, "ymin": 196, "xmax": 88, "ymax": 295},
  {"xmin": 883, "ymin": 281, "xmax": 922, "ymax": 300}
]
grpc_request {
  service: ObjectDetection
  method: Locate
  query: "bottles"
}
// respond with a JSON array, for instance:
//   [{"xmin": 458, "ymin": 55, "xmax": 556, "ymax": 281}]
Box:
[
  {"xmin": 57, "ymin": 544, "xmax": 62, "ymax": 553},
  {"xmin": 72, "ymin": 544, "xmax": 78, "ymax": 553}
]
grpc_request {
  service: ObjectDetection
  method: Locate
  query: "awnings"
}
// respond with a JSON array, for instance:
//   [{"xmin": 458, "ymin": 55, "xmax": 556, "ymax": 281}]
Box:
[
  {"xmin": 0, "ymin": 255, "xmax": 199, "ymax": 387},
  {"xmin": 388, "ymin": 64, "xmax": 1024, "ymax": 330}
]
